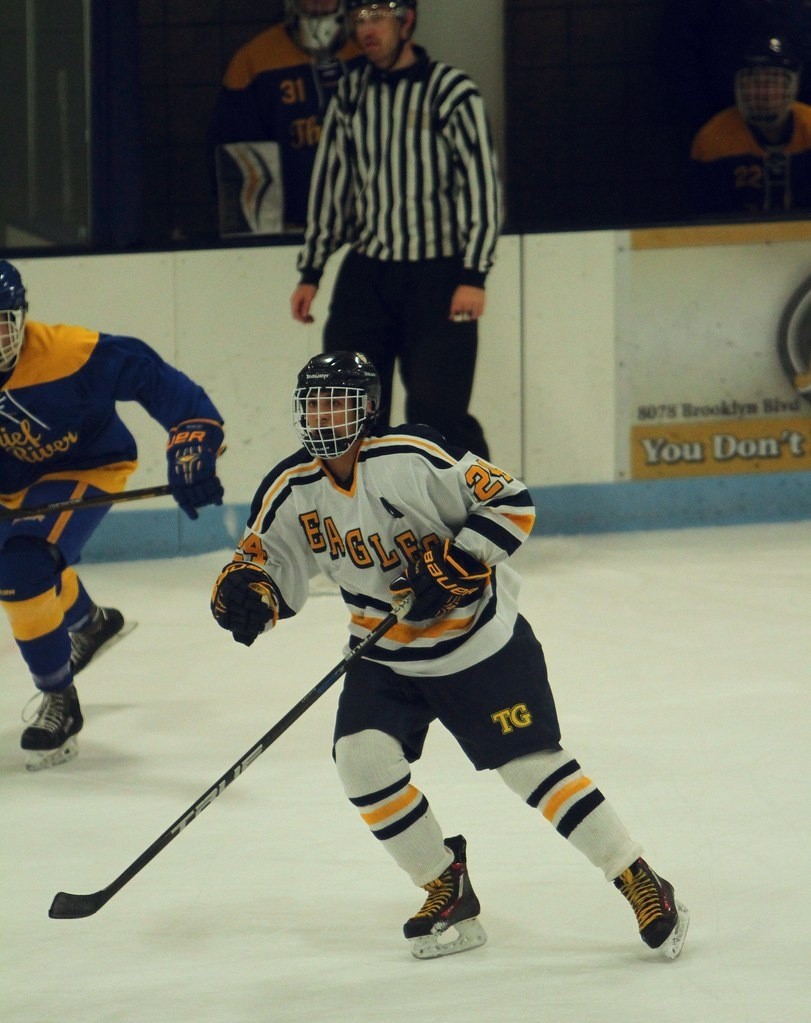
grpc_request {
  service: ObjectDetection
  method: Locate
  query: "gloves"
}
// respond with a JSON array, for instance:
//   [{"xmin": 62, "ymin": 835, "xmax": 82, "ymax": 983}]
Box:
[
  {"xmin": 389, "ymin": 537, "xmax": 492, "ymax": 626},
  {"xmin": 167, "ymin": 419, "xmax": 224, "ymax": 519},
  {"xmin": 210, "ymin": 563, "xmax": 276, "ymax": 647}
]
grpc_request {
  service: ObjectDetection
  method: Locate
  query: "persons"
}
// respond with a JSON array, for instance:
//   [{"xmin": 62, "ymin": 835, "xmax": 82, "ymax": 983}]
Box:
[
  {"xmin": 0, "ymin": 258, "xmax": 226, "ymax": 771},
  {"xmin": 288, "ymin": 0, "xmax": 499, "ymax": 463},
  {"xmin": 210, "ymin": 349, "xmax": 690, "ymax": 961},
  {"xmin": 680, "ymin": 21, "xmax": 811, "ymax": 224},
  {"xmin": 223, "ymin": 0, "xmax": 370, "ymax": 234}
]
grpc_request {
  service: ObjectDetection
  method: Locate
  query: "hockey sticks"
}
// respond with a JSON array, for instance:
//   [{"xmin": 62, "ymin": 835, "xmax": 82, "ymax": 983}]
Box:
[
  {"xmin": 5, "ymin": 484, "xmax": 173, "ymax": 520},
  {"xmin": 45, "ymin": 591, "xmax": 415, "ymax": 919}
]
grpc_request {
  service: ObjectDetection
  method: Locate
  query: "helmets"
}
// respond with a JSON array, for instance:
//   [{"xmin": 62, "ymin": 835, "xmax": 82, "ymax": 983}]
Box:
[
  {"xmin": 734, "ymin": 36, "xmax": 797, "ymax": 127},
  {"xmin": 293, "ymin": 349, "xmax": 381, "ymax": 460},
  {"xmin": 0, "ymin": 260, "xmax": 28, "ymax": 387},
  {"xmin": 345, "ymin": 0, "xmax": 416, "ymax": 17},
  {"xmin": 286, "ymin": 0, "xmax": 346, "ymax": 48}
]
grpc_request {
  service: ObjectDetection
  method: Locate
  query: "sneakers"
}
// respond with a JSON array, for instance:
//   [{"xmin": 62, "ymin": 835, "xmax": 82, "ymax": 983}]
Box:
[
  {"xmin": 612, "ymin": 856, "xmax": 690, "ymax": 959},
  {"xmin": 67, "ymin": 602, "xmax": 137, "ymax": 681},
  {"xmin": 402, "ymin": 835, "xmax": 486, "ymax": 959},
  {"xmin": 20, "ymin": 682, "xmax": 84, "ymax": 771}
]
